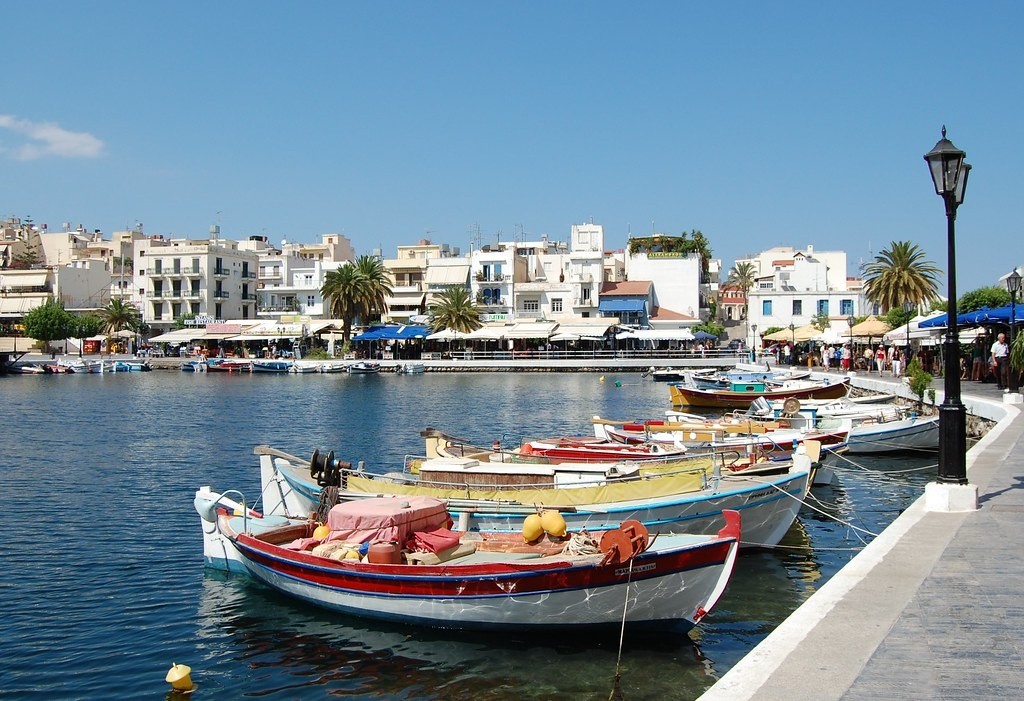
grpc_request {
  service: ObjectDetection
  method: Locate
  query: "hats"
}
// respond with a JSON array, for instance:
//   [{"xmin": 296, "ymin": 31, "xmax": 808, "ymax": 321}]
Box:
[{"xmin": 879, "ymin": 345, "xmax": 883, "ymax": 348}]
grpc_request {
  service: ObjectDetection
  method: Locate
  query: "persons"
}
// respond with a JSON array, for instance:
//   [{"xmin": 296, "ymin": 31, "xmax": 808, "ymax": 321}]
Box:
[
  {"xmin": 109, "ymin": 340, "xmax": 281, "ymax": 358},
  {"xmin": 970, "ymin": 338, "xmax": 984, "ymax": 381},
  {"xmin": 959, "ymin": 352, "xmax": 969, "ymax": 380},
  {"xmin": 628, "ymin": 339, "xmax": 712, "ymax": 358},
  {"xmin": 390, "ymin": 339, "xmax": 499, "ymax": 360},
  {"xmin": 736, "ymin": 337, "xmax": 746, "ymax": 358},
  {"xmin": 767, "ymin": 335, "xmax": 911, "ymax": 390},
  {"xmin": 987, "ymin": 333, "xmax": 1011, "ymax": 390},
  {"xmin": 526, "ymin": 343, "xmax": 560, "ymax": 351}
]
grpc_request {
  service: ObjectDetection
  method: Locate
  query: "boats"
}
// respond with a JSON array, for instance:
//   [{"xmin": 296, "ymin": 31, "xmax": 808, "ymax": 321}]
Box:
[
  {"xmin": 402, "ymin": 363, "xmax": 425, "ymax": 373},
  {"xmin": 347, "ymin": 362, "xmax": 380, "ymax": 374},
  {"xmin": 194, "ymin": 486, "xmax": 742, "ymax": 639},
  {"xmin": 254, "ymin": 393, "xmax": 941, "ymax": 552},
  {"xmin": 181, "ymin": 354, "xmax": 289, "ymax": 373},
  {"xmin": 641, "ymin": 366, "xmax": 851, "ymax": 408},
  {"xmin": 315, "ymin": 362, "xmax": 347, "ymax": 373},
  {"xmin": 10, "ymin": 360, "xmax": 145, "ymax": 374},
  {"xmin": 288, "ymin": 359, "xmax": 316, "ymax": 373}
]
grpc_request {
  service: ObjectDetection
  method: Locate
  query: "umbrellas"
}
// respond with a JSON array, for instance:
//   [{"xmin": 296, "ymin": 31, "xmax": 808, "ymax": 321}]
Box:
[
  {"xmin": 463, "ymin": 325, "xmax": 504, "ymax": 340},
  {"xmin": 549, "ymin": 333, "xmax": 580, "ymax": 341},
  {"xmin": 615, "ymin": 331, "xmax": 638, "ymax": 340},
  {"xmin": 918, "ymin": 301, "xmax": 1024, "ymax": 330},
  {"xmin": 580, "ymin": 336, "xmax": 607, "ymax": 341},
  {"xmin": 794, "ymin": 325, "xmax": 820, "ymax": 344},
  {"xmin": 842, "ymin": 316, "xmax": 895, "ymax": 341},
  {"xmin": 693, "ymin": 330, "xmax": 718, "ymax": 340},
  {"xmin": 762, "ymin": 327, "xmax": 793, "ymax": 344},
  {"xmin": 426, "ymin": 327, "xmax": 467, "ymax": 342},
  {"xmin": 811, "ymin": 329, "xmax": 847, "ymax": 346},
  {"xmin": 883, "ymin": 315, "xmax": 926, "ymax": 341},
  {"xmin": 909, "ymin": 309, "xmax": 986, "ymax": 339}
]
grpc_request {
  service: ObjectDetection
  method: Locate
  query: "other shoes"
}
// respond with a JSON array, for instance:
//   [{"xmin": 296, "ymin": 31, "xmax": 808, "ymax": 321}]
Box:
[{"xmin": 998, "ymin": 385, "xmax": 1007, "ymax": 390}]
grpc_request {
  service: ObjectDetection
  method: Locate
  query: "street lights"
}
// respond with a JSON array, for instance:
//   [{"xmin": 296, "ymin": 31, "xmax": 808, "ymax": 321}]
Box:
[
  {"xmin": 847, "ymin": 313, "xmax": 856, "ymax": 377},
  {"xmin": 902, "ymin": 300, "xmax": 914, "ymax": 385},
  {"xmin": 925, "ymin": 124, "xmax": 981, "ymax": 511},
  {"xmin": 788, "ymin": 322, "xmax": 797, "ymax": 371},
  {"xmin": 751, "ymin": 323, "xmax": 757, "ymax": 366},
  {"xmin": 1003, "ymin": 267, "xmax": 1023, "ymax": 405}
]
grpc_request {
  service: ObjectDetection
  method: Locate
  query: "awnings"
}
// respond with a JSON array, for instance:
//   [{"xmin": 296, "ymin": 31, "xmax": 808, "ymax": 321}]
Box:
[
  {"xmin": 352, "ymin": 325, "xmax": 430, "ymax": 340},
  {"xmin": 634, "ymin": 328, "xmax": 695, "ymax": 341},
  {"xmin": 505, "ymin": 321, "xmax": 558, "ymax": 339},
  {"xmin": 552, "ymin": 325, "xmax": 611, "ymax": 339}
]
